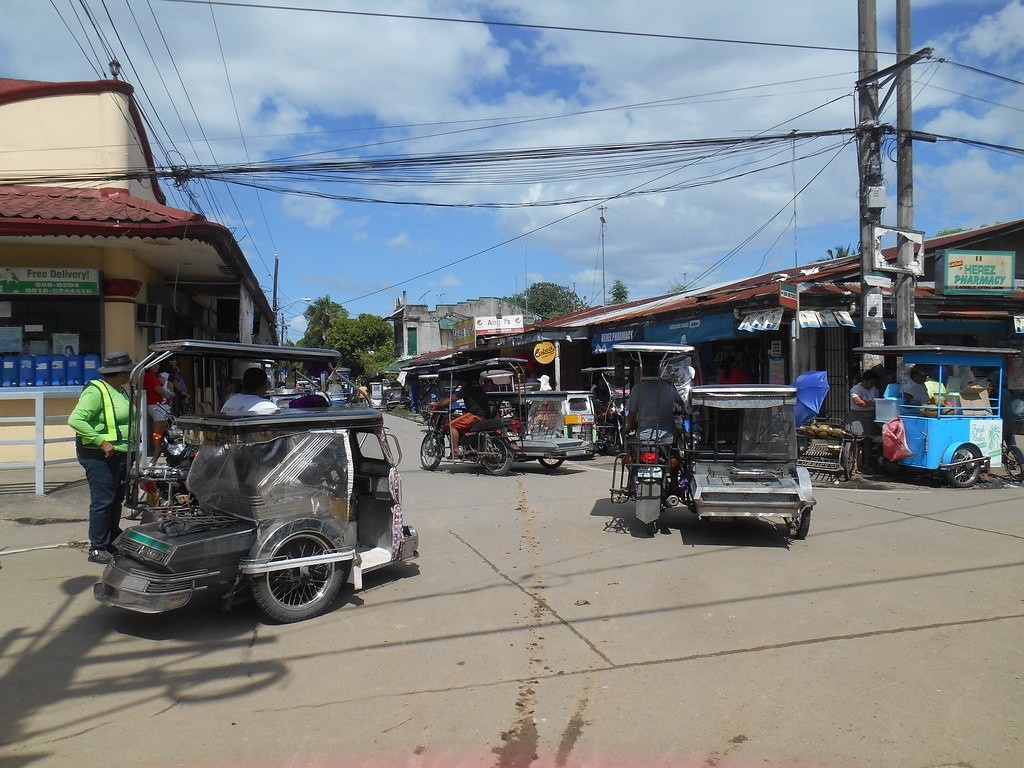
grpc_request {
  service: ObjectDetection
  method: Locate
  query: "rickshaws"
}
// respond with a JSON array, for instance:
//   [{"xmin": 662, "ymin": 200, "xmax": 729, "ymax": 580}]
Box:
[
  {"xmin": 419, "ymin": 358, "xmax": 594, "ymax": 475},
  {"xmin": 92, "ymin": 340, "xmax": 420, "ymax": 624},
  {"xmin": 579, "ymin": 341, "xmax": 696, "ymax": 455},
  {"xmin": 610, "ymin": 342, "xmax": 817, "ymax": 540}
]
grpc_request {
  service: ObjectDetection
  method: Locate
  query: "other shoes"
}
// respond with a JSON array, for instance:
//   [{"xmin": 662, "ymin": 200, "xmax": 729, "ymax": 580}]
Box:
[
  {"xmin": 89, "ymin": 549, "xmax": 113, "ymax": 565},
  {"xmin": 449, "ymin": 451, "xmax": 463, "ymax": 460},
  {"xmin": 672, "ymin": 485, "xmax": 684, "ymax": 495}
]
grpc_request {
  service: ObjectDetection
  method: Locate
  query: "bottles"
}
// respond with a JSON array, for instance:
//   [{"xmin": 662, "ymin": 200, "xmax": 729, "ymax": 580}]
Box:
[{"xmin": 635, "ymin": 452, "xmax": 662, "ymax": 524}]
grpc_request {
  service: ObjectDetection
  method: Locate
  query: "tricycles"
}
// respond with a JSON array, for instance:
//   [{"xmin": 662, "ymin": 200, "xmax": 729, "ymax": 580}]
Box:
[{"xmin": 771, "ymin": 408, "xmax": 869, "ymax": 481}]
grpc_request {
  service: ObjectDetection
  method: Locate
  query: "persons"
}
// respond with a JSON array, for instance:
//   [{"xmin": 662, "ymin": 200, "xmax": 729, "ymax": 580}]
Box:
[
  {"xmin": 157, "ymin": 371, "xmax": 169, "ymax": 405},
  {"xmin": 169, "ymin": 358, "xmax": 192, "ymax": 417},
  {"xmin": 295, "ymin": 383, "xmax": 302, "ymax": 388},
  {"xmin": 431, "ymin": 371, "xmax": 491, "ymax": 457},
  {"xmin": 715, "ymin": 354, "xmax": 750, "ymax": 433},
  {"xmin": 145, "ymin": 356, "xmax": 175, "ymax": 453},
  {"xmin": 65, "ymin": 345, "xmax": 75, "ymax": 355},
  {"xmin": 621, "ymin": 359, "xmax": 690, "ymax": 494},
  {"xmin": 593, "ymin": 373, "xmax": 613, "ymax": 438},
  {"xmin": 849, "ymin": 371, "xmax": 880, "ymax": 473},
  {"xmin": 68, "ymin": 351, "xmax": 139, "ymax": 564},
  {"xmin": 530, "ymin": 374, "xmax": 552, "ymax": 391},
  {"xmin": 220, "ymin": 368, "xmax": 280, "ymax": 412},
  {"xmin": 988, "ymin": 369, "xmax": 1024, "ymax": 478},
  {"xmin": 900, "ymin": 365, "xmax": 931, "ymax": 404},
  {"xmin": 358, "ymin": 382, "xmax": 368, "ymax": 404}
]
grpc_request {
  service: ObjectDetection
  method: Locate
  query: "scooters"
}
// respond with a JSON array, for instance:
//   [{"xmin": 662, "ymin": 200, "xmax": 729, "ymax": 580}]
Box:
[{"xmin": 1002, "ymin": 420, "xmax": 1024, "ymax": 482}]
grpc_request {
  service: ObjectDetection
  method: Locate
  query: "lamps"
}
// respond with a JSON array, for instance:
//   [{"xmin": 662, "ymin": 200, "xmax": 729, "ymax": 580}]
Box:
[{"xmin": 108, "ymin": 58, "xmax": 122, "ymax": 81}]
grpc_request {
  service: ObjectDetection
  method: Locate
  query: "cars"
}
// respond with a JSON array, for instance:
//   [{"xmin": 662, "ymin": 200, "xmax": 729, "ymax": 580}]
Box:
[{"xmin": 262, "ymin": 367, "xmax": 368, "ymax": 408}]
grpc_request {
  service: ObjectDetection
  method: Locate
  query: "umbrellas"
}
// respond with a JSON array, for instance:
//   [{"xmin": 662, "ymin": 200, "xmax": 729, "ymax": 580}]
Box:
[{"xmin": 790, "ymin": 369, "xmax": 832, "ymax": 427}]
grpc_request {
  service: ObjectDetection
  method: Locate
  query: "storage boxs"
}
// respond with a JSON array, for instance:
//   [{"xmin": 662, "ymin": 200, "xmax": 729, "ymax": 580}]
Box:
[{"xmin": 874, "ymin": 383, "xmax": 905, "ymax": 421}]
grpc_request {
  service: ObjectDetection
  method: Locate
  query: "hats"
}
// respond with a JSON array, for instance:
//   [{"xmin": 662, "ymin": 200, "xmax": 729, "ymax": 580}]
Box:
[{"xmin": 97, "ymin": 352, "xmax": 137, "ymax": 374}]
